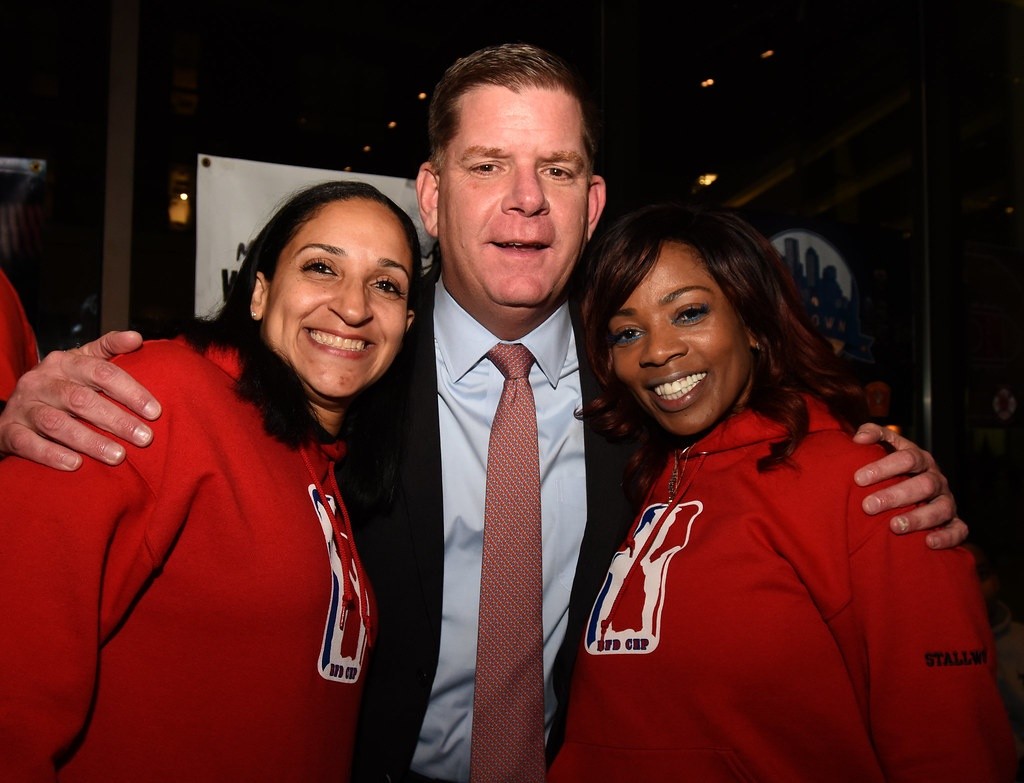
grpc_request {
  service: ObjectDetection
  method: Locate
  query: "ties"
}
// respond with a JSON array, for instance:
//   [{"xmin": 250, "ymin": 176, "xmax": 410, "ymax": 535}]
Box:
[{"xmin": 470, "ymin": 344, "xmax": 546, "ymax": 783}]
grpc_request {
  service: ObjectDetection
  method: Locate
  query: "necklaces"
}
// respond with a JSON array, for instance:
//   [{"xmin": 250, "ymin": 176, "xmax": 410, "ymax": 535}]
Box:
[{"xmin": 668, "ymin": 441, "xmax": 696, "ymax": 502}]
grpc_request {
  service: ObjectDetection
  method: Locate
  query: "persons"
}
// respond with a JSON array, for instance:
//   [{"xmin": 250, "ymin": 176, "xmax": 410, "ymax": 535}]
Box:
[
  {"xmin": 0, "ymin": 181, "xmax": 422, "ymax": 783},
  {"xmin": 548, "ymin": 205, "xmax": 1017, "ymax": 783},
  {"xmin": 1, "ymin": 43, "xmax": 969, "ymax": 783}
]
